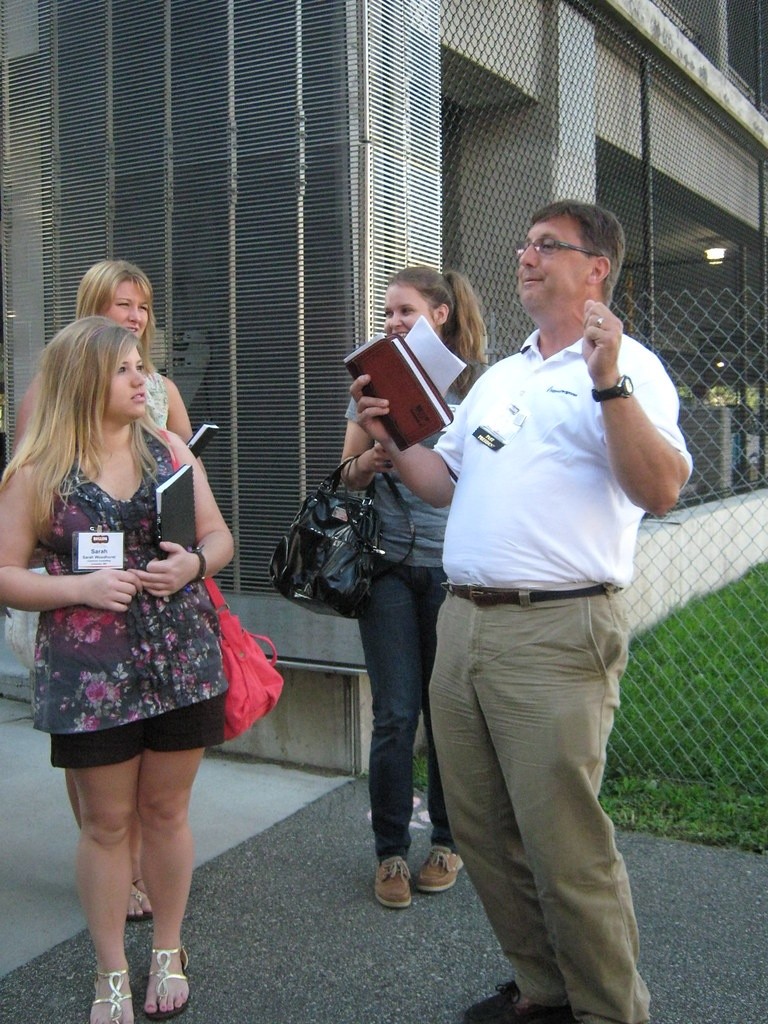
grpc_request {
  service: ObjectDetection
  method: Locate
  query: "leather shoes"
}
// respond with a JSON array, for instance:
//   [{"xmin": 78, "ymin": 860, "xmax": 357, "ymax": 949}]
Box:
[
  {"xmin": 375, "ymin": 856, "xmax": 411, "ymax": 908},
  {"xmin": 414, "ymin": 845, "xmax": 464, "ymax": 894},
  {"xmin": 463, "ymin": 978, "xmax": 575, "ymax": 1024}
]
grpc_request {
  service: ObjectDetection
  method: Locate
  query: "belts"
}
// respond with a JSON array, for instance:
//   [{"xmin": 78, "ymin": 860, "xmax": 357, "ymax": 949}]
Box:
[{"xmin": 446, "ymin": 579, "xmax": 624, "ymax": 608}]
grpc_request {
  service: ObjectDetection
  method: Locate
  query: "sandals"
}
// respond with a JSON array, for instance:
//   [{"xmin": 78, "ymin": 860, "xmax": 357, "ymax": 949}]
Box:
[{"xmin": 90, "ymin": 942, "xmax": 189, "ymax": 1024}]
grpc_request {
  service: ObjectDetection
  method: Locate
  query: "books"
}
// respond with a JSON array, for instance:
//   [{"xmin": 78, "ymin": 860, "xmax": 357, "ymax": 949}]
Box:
[
  {"xmin": 344, "ymin": 332, "xmax": 455, "ymax": 453},
  {"xmin": 187, "ymin": 421, "xmax": 220, "ymax": 459},
  {"xmin": 154, "ymin": 463, "xmax": 197, "ymax": 560}
]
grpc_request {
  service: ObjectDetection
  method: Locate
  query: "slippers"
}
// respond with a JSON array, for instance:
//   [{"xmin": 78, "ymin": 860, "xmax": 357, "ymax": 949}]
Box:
[{"xmin": 123, "ymin": 875, "xmax": 155, "ymax": 923}]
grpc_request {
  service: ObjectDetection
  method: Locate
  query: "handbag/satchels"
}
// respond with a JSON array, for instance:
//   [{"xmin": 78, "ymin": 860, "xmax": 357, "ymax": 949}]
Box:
[
  {"xmin": 201, "ymin": 576, "xmax": 285, "ymax": 744},
  {"xmin": 269, "ymin": 453, "xmax": 387, "ymax": 619}
]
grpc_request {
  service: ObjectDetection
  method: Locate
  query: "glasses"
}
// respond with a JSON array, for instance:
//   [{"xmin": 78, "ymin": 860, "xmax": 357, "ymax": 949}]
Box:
[{"xmin": 514, "ymin": 236, "xmax": 605, "ymax": 261}]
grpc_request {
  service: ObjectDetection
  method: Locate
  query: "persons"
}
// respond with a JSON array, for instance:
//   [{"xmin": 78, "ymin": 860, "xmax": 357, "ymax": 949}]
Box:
[
  {"xmin": 0, "ymin": 316, "xmax": 238, "ymax": 1024},
  {"xmin": 335, "ymin": 264, "xmax": 494, "ymax": 909},
  {"xmin": 12, "ymin": 259, "xmax": 209, "ymax": 481},
  {"xmin": 347, "ymin": 198, "xmax": 697, "ymax": 1024}
]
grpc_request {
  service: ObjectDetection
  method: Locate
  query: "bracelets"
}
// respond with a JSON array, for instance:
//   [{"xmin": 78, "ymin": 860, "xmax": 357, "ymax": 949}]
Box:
[{"xmin": 192, "ymin": 544, "xmax": 207, "ymax": 583}]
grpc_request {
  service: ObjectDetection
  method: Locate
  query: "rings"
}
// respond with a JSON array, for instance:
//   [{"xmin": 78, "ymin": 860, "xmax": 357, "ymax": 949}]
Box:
[{"xmin": 595, "ymin": 317, "xmax": 605, "ymax": 329}]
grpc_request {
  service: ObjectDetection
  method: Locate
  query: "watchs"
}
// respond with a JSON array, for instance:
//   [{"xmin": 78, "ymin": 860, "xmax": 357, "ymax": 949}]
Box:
[{"xmin": 592, "ymin": 373, "xmax": 636, "ymax": 403}]
